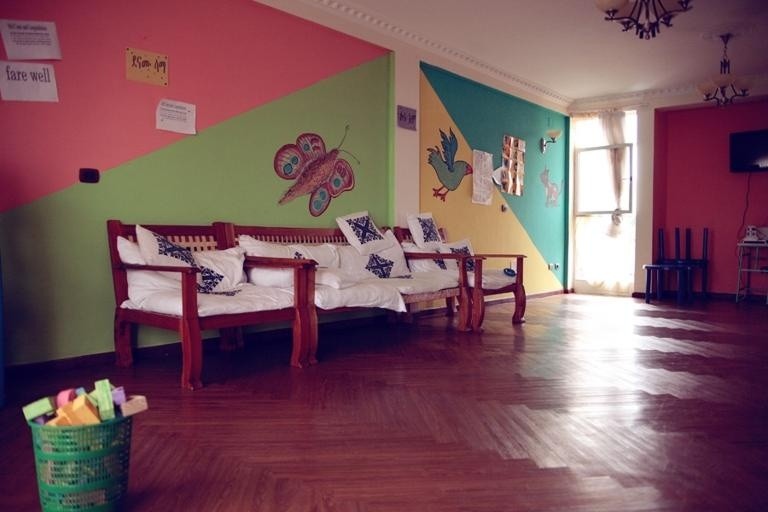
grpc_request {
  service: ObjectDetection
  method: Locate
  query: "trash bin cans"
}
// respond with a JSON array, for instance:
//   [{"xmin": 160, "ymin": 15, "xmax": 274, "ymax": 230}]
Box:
[{"xmin": 27, "ymin": 415, "xmax": 133, "ymax": 512}]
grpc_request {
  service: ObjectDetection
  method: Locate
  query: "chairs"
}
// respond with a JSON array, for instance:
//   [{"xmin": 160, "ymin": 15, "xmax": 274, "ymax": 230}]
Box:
[
  {"xmin": 391, "ymin": 224, "xmax": 528, "ymax": 336},
  {"xmin": 102, "ymin": 217, "xmax": 308, "ymax": 389}
]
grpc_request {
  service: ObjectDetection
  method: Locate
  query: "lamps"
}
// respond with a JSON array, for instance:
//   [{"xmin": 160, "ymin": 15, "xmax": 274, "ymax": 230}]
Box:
[
  {"xmin": 594, "ymin": 0, "xmax": 695, "ymax": 43},
  {"xmin": 697, "ymin": 32, "xmax": 758, "ymax": 109},
  {"xmin": 538, "ymin": 128, "xmax": 562, "ymax": 154}
]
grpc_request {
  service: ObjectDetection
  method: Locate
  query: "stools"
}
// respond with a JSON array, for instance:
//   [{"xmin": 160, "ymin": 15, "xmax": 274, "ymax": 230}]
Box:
[{"xmin": 641, "ymin": 226, "xmax": 710, "ymax": 307}]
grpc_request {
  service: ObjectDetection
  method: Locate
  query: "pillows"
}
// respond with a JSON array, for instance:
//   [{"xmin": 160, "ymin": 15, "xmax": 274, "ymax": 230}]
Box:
[
  {"xmin": 238, "ymin": 208, "xmax": 478, "ymax": 284},
  {"xmin": 115, "ymin": 223, "xmax": 247, "ymax": 307}
]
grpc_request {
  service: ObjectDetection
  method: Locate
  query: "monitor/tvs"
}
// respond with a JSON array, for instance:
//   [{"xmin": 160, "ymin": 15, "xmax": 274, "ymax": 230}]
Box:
[{"xmin": 729, "ymin": 128, "xmax": 768, "ymax": 173}]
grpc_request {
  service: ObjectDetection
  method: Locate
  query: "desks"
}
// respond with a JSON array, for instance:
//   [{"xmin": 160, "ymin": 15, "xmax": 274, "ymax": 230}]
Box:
[{"xmin": 734, "ymin": 240, "xmax": 767, "ymax": 306}]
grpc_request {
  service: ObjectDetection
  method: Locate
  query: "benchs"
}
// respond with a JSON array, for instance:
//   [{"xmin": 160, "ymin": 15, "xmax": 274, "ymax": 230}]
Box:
[{"xmin": 224, "ymin": 220, "xmax": 476, "ymax": 369}]
